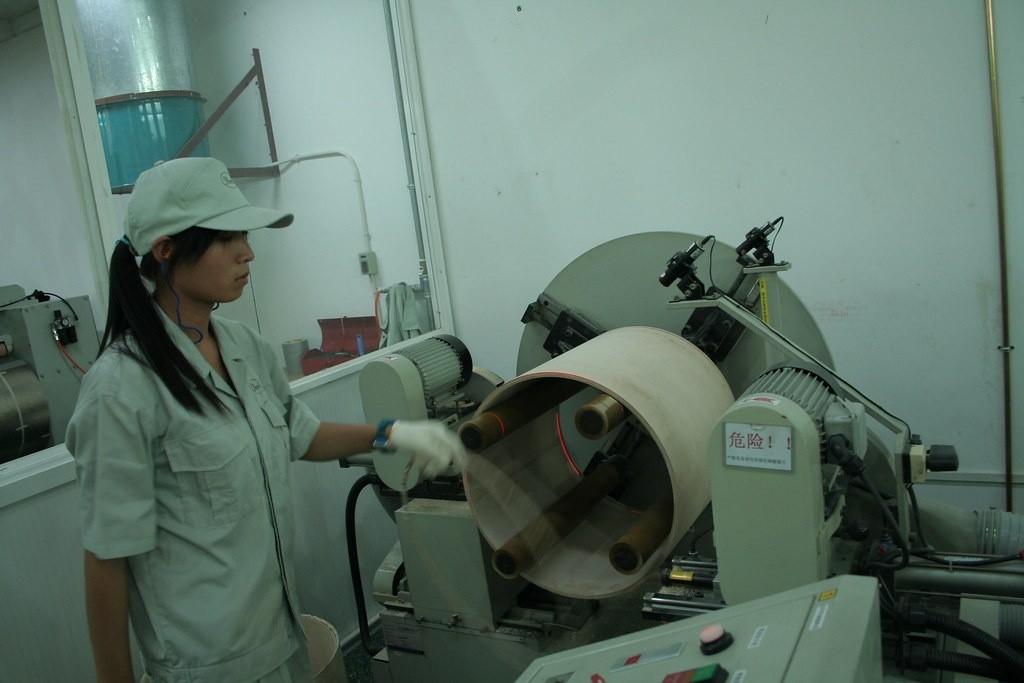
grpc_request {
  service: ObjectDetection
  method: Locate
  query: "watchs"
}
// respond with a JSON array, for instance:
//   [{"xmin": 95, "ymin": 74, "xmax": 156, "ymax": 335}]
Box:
[{"xmin": 374, "ymin": 419, "xmax": 397, "ymax": 454}]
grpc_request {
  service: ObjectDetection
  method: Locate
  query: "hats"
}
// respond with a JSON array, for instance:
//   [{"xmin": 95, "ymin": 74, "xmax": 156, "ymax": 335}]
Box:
[{"xmin": 122, "ymin": 156, "xmax": 294, "ymax": 257}]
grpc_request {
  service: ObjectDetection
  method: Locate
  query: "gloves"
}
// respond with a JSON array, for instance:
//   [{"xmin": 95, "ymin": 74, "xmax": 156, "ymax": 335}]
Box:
[{"xmin": 389, "ymin": 419, "xmax": 468, "ymax": 480}]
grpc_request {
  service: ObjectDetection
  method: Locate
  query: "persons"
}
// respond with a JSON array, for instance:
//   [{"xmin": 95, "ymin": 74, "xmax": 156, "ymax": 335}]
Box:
[{"xmin": 65, "ymin": 157, "xmax": 464, "ymax": 683}]
